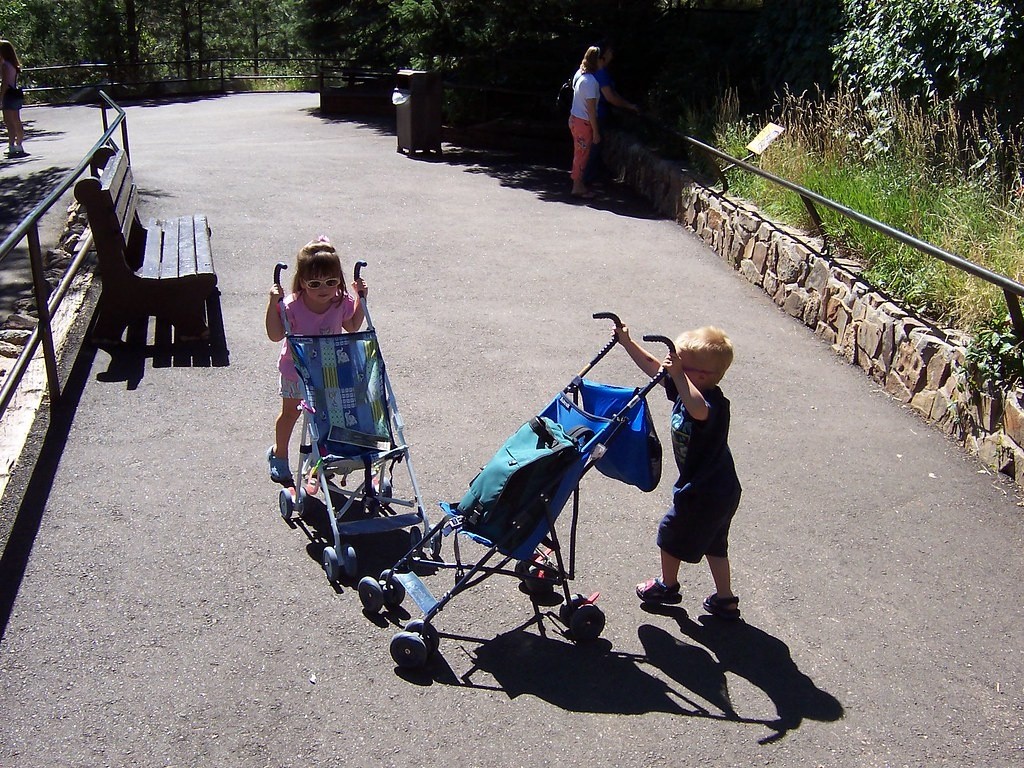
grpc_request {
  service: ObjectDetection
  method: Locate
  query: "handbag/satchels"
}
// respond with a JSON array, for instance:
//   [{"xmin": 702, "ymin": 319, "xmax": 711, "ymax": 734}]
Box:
[{"xmin": 556, "ymin": 75, "xmax": 574, "ymax": 111}]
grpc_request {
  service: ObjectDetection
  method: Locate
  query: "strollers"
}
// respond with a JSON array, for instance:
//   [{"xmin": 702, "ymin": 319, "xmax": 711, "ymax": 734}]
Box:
[
  {"xmin": 272, "ymin": 259, "xmax": 443, "ymax": 583},
  {"xmin": 357, "ymin": 310, "xmax": 679, "ymax": 674}
]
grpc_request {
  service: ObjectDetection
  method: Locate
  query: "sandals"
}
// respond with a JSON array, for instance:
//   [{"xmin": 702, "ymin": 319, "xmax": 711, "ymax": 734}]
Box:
[
  {"xmin": 266, "ymin": 444, "xmax": 292, "ymax": 482},
  {"xmin": 636, "ymin": 578, "xmax": 682, "ymax": 604},
  {"xmin": 703, "ymin": 593, "xmax": 741, "ymax": 619}
]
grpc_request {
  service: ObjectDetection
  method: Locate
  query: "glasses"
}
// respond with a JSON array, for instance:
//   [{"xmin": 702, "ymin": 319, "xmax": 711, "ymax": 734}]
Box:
[{"xmin": 302, "ymin": 278, "xmax": 340, "ymax": 289}]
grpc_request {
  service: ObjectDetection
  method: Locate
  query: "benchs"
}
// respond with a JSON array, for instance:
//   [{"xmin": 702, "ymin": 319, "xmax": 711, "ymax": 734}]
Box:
[{"xmin": 74, "ymin": 146, "xmax": 227, "ymax": 351}]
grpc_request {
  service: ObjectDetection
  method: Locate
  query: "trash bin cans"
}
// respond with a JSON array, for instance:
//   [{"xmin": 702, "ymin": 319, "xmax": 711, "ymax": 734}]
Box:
[{"xmin": 393, "ymin": 69, "xmax": 442, "ymax": 156}]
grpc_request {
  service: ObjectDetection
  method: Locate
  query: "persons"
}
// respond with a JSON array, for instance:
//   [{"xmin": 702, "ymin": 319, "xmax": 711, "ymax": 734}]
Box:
[
  {"xmin": 0, "ymin": 39, "xmax": 23, "ymax": 152},
  {"xmin": 266, "ymin": 236, "xmax": 369, "ymax": 481},
  {"xmin": 610, "ymin": 323, "xmax": 742, "ymax": 618},
  {"xmin": 567, "ymin": 36, "xmax": 641, "ymax": 199}
]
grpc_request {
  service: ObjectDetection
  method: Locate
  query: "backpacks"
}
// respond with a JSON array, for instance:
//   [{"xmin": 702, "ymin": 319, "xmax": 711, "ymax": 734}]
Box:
[{"xmin": 458, "ymin": 415, "xmax": 595, "ymax": 536}]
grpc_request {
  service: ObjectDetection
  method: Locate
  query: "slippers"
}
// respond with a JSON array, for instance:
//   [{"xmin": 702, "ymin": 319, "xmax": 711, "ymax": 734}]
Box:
[{"xmin": 572, "ymin": 190, "xmax": 595, "ymax": 199}]
